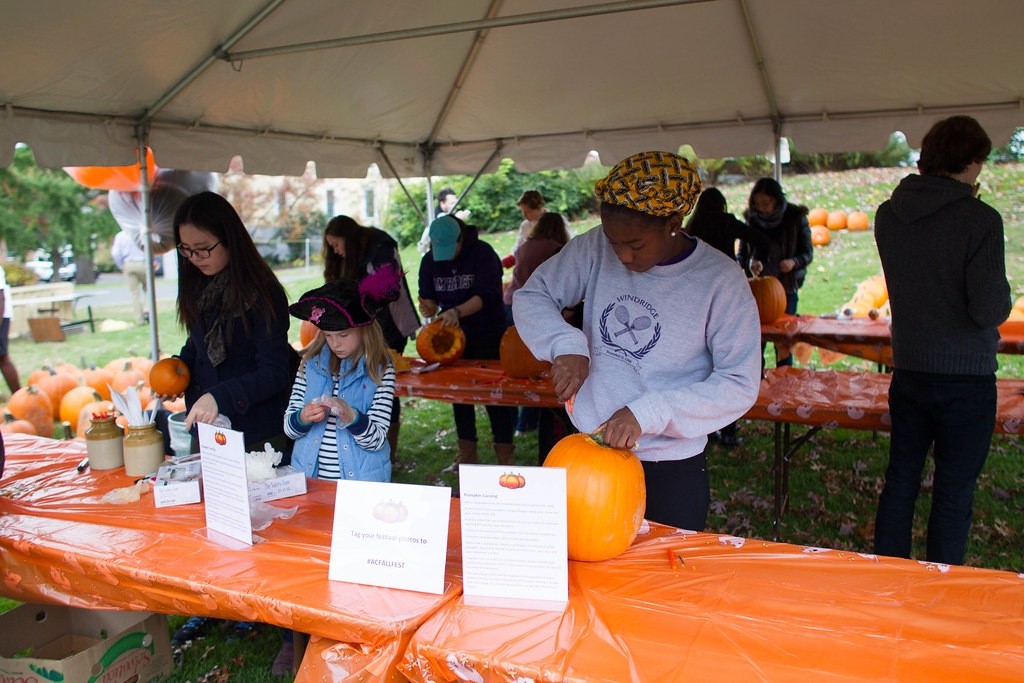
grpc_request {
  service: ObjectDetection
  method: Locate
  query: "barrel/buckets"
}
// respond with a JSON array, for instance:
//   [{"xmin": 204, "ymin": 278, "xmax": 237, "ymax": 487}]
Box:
[{"xmin": 168, "ymin": 411, "xmax": 191, "ymax": 458}]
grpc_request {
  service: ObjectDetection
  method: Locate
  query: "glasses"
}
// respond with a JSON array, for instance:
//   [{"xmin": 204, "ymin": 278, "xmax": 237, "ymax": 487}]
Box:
[
  {"xmin": 176, "ymin": 241, "xmax": 220, "ymax": 259},
  {"xmin": 752, "ymin": 200, "xmax": 772, "ymax": 208}
]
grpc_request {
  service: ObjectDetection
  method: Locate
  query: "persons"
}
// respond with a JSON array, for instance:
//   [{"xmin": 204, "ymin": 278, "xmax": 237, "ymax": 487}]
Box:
[
  {"xmin": 684, "ymin": 177, "xmax": 813, "ymax": 446},
  {"xmin": 499, "ymin": 189, "xmax": 573, "ymax": 437},
  {"xmin": 511, "ymin": 150, "xmax": 763, "ymax": 532},
  {"xmin": 871, "ymin": 116, "xmax": 1015, "ymax": 565},
  {"xmin": 321, "ymin": 215, "xmax": 424, "ymax": 472},
  {"xmin": 417, "ymin": 215, "xmax": 520, "ymax": 477},
  {"xmin": 433, "ymin": 188, "xmax": 460, "ymax": 219},
  {"xmin": 110, "ymin": 230, "xmax": 148, "ymax": 326},
  {"xmin": 0, "ymin": 265, "xmax": 22, "ymax": 394},
  {"xmin": 149, "ymin": 191, "xmax": 292, "ymax": 643},
  {"xmin": 270, "ymin": 265, "xmax": 408, "ymax": 677}
]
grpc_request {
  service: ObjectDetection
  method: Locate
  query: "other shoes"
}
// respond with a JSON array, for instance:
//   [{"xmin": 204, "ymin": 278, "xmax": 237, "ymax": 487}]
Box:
[{"xmin": 707, "ymin": 424, "xmax": 746, "ymax": 448}]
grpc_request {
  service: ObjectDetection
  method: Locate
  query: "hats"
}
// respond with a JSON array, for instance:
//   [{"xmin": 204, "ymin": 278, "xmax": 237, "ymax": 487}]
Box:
[
  {"xmin": 288, "ymin": 262, "xmax": 409, "ymax": 332},
  {"xmin": 429, "ymin": 216, "xmax": 460, "ymax": 262},
  {"xmin": 595, "ymin": 151, "xmax": 702, "ymax": 216}
]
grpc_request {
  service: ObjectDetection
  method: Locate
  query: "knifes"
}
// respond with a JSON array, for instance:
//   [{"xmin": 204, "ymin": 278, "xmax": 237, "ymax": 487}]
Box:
[{"xmin": 566, "ymin": 398, "xmax": 581, "ymax": 433}]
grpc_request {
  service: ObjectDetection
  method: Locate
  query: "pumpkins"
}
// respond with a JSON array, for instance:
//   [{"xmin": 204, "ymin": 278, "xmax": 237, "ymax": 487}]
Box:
[
  {"xmin": 300, "ymin": 320, "xmax": 319, "ymax": 348},
  {"xmin": 0, "ymin": 350, "xmax": 190, "ymax": 441},
  {"xmin": 500, "ymin": 325, "xmax": 551, "ymax": 379},
  {"xmin": 807, "ymin": 207, "xmax": 868, "ymax": 244},
  {"xmin": 840, "ymin": 275, "xmax": 891, "ymax": 317},
  {"xmin": 1009, "ymin": 296, "xmax": 1024, "ymax": 322},
  {"xmin": 541, "ymin": 433, "xmax": 646, "ymax": 561}
]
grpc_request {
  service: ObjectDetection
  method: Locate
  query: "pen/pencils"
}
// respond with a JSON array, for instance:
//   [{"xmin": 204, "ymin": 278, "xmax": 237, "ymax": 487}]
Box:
[{"xmin": 134, "ymin": 472, "xmax": 156, "ymax": 484}]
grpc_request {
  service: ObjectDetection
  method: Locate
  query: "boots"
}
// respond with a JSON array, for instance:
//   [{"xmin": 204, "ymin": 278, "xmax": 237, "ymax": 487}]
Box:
[
  {"xmin": 493, "ymin": 443, "xmax": 516, "ymax": 465},
  {"xmin": 442, "ymin": 440, "xmax": 477, "ymax": 473}
]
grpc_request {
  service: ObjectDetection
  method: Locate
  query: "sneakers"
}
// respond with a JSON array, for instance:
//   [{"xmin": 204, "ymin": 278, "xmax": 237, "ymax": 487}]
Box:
[
  {"xmin": 172, "ymin": 616, "xmax": 226, "ymax": 641},
  {"xmin": 272, "ymin": 643, "xmax": 295, "ymax": 674},
  {"xmin": 233, "ymin": 621, "xmax": 267, "ymax": 635}
]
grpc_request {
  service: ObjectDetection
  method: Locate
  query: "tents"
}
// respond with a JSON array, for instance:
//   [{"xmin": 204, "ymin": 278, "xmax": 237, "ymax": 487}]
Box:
[{"xmin": 0, "ymin": 0, "xmax": 1024, "ymax": 414}]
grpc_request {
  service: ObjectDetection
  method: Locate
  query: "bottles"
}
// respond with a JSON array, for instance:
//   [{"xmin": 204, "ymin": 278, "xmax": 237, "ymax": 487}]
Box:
[
  {"xmin": 123, "ymin": 421, "xmax": 163, "ymax": 475},
  {"xmin": 85, "ymin": 415, "xmax": 124, "ymax": 470}
]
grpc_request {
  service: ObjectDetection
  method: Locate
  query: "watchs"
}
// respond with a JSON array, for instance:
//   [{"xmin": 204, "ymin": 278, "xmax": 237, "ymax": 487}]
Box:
[{"xmin": 451, "ymin": 306, "xmax": 462, "ymax": 319}]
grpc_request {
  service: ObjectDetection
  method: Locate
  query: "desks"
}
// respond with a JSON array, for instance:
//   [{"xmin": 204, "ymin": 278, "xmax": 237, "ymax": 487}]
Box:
[{"xmin": 0, "ymin": 311, "xmax": 1024, "ymax": 683}]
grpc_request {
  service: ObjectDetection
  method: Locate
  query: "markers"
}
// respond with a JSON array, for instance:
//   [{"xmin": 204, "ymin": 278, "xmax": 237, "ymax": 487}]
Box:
[{"xmin": 76, "ymin": 458, "xmax": 89, "ymax": 473}]
